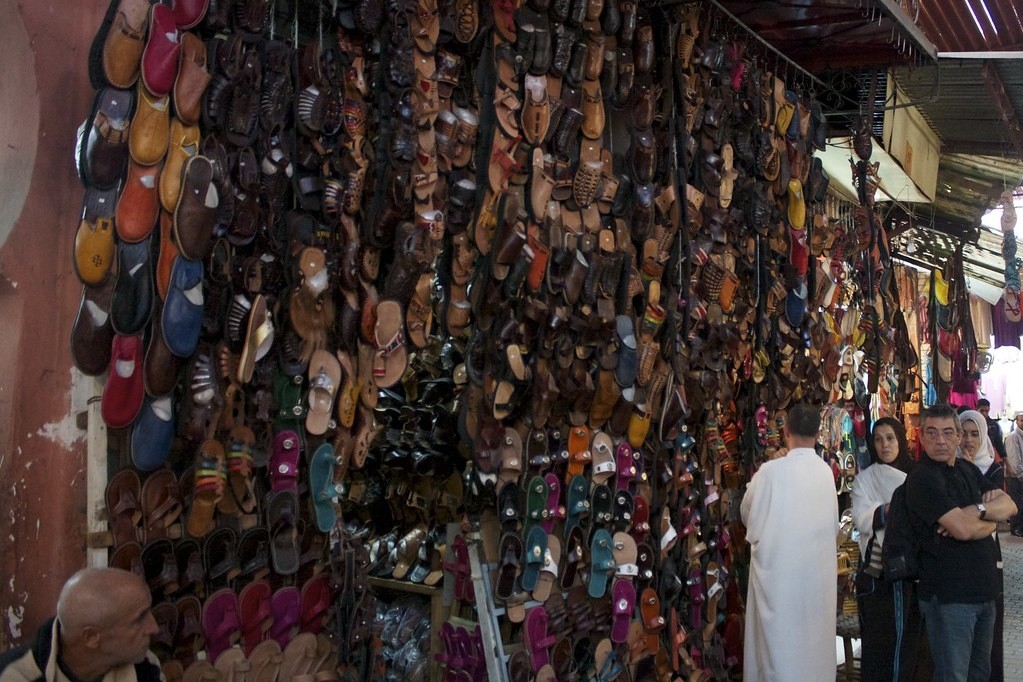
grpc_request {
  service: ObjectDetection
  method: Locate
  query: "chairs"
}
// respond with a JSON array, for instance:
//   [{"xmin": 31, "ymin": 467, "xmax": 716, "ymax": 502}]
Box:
[{"xmin": 836, "ymin": 551, "xmax": 862, "ymax": 682}]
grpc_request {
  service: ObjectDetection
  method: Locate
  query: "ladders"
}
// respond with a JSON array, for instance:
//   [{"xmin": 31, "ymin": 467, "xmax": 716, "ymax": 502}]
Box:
[{"xmin": 466, "ymin": 528, "xmax": 570, "ymax": 682}]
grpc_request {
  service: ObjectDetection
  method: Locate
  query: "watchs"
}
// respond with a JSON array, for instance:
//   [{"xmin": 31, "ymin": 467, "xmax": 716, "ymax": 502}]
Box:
[{"xmin": 976, "ymin": 501, "xmax": 987, "ymax": 522}]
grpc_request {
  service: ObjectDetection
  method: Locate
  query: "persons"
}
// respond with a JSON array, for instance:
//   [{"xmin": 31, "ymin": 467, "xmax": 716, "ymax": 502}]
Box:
[
  {"xmin": 1, "ymin": 565, "xmax": 169, "ymax": 682},
  {"xmin": 955, "ymin": 408, "xmax": 1009, "ymax": 682},
  {"xmin": 897, "ymin": 403, "xmax": 1019, "ymax": 681},
  {"xmin": 1002, "ymin": 410, "xmax": 1023, "ymax": 539},
  {"xmin": 849, "ymin": 414, "xmax": 927, "ymax": 681},
  {"xmin": 740, "ymin": 402, "xmax": 839, "ymax": 681},
  {"xmin": 975, "ymin": 397, "xmax": 1006, "ymax": 460}
]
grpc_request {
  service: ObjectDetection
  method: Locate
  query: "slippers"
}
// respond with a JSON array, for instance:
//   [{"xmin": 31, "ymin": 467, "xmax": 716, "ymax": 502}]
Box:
[{"xmin": 75, "ymin": 0, "xmax": 960, "ymax": 681}]
